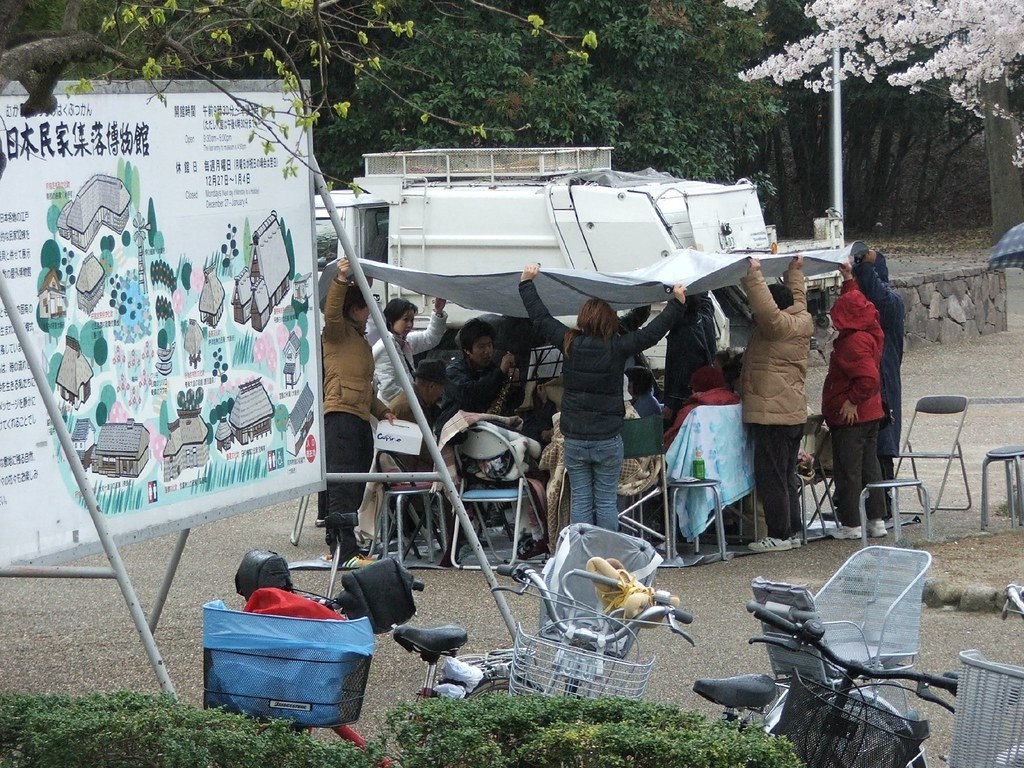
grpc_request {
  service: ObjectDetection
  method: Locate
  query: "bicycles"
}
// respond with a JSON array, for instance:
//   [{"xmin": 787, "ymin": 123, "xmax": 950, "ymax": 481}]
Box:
[
  {"xmin": 200, "ymin": 546, "xmax": 552, "ymax": 768},
  {"xmin": 689, "ymin": 545, "xmax": 1023, "ymax": 768},
  {"xmin": 493, "ymin": 520, "xmax": 698, "ymax": 709}
]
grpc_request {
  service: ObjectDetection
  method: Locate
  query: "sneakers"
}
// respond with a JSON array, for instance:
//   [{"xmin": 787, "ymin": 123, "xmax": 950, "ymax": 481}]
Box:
[
  {"xmin": 787, "ymin": 534, "xmax": 802, "ymax": 548},
  {"xmin": 749, "ymin": 536, "xmax": 792, "ymax": 551},
  {"xmin": 828, "ymin": 525, "xmax": 863, "ymax": 540},
  {"xmin": 338, "ymin": 554, "xmax": 375, "ymax": 568},
  {"xmin": 864, "ymin": 520, "xmax": 888, "ymax": 537}
]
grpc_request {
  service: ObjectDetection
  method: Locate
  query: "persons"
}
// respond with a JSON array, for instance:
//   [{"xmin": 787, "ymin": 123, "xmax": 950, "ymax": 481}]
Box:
[
  {"xmin": 822, "ymin": 248, "xmax": 905, "ymax": 530},
  {"xmin": 740, "ymin": 255, "xmax": 815, "ymax": 549},
  {"xmin": 372, "ymin": 296, "xmax": 448, "ymax": 406},
  {"xmin": 621, "ymin": 350, "xmax": 740, "ymax": 555},
  {"xmin": 316, "ymin": 276, "xmax": 387, "ymax": 526},
  {"xmin": 389, "ymin": 319, "xmax": 554, "ymax": 546},
  {"xmin": 822, "ymin": 261, "xmax": 888, "ymax": 539},
  {"xmin": 519, "ymin": 265, "xmax": 715, "ymax": 532},
  {"xmin": 320, "ymin": 259, "xmax": 397, "ymax": 568}
]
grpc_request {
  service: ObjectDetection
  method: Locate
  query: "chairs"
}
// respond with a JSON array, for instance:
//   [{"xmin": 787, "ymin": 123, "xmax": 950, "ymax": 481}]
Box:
[
  {"xmin": 811, "ymin": 546, "xmax": 932, "ymax": 679},
  {"xmin": 799, "ymin": 414, "xmax": 840, "ymax": 543},
  {"xmin": 550, "ymin": 415, "xmax": 669, "ymax": 559},
  {"xmin": 449, "ymin": 427, "xmax": 546, "ymax": 569},
  {"xmin": 538, "ymin": 523, "xmax": 664, "ymax": 661},
  {"xmin": 900, "ymin": 396, "xmax": 972, "ymax": 515}
]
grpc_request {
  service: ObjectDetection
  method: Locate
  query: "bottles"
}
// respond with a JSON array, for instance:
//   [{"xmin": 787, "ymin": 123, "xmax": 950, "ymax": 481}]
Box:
[{"xmin": 693, "ymin": 451, "xmax": 705, "ymax": 480}]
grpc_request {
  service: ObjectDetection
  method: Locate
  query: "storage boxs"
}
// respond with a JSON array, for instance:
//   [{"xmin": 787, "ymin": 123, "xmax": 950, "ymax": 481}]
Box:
[{"xmin": 375, "ymin": 420, "xmax": 423, "ymax": 455}]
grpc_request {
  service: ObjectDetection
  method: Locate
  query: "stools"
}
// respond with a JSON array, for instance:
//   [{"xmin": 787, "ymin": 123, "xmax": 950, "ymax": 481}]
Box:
[
  {"xmin": 859, "ymin": 478, "xmax": 932, "ymax": 551},
  {"xmin": 981, "ymin": 447, "xmax": 1024, "ymax": 529},
  {"xmin": 668, "ymin": 479, "xmax": 728, "ymax": 562},
  {"xmin": 380, "ymin": 481, "xmax": 451, "ymax": 564}
]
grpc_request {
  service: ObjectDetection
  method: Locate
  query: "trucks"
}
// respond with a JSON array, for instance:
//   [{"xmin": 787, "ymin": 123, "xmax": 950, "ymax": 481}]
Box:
[{"xmin": 315, "ymin": 145, "xmax": 776, "ymax": 394}]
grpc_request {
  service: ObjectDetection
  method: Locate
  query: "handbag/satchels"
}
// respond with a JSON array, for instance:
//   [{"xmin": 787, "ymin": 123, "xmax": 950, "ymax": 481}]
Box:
[{"xmin": 879, "ymin": 400, "xmax": 892, "ymax": 431}]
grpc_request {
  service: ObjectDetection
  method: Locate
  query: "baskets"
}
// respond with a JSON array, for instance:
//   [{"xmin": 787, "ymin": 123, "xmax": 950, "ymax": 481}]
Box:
[
  {"xmin": 774, "ymin": 664, "xmax": 930, "ymax": 768},
  {"xmin": 949, "ymin": 649, "xmax": 1024, "ymax": 768},
  {"xmin": 203, "ymin": 600, "xmax": 373, "ymax": 728},
  {"xmin": 511, "ymin": 621, "xmax": 657, "ymax": 700}
]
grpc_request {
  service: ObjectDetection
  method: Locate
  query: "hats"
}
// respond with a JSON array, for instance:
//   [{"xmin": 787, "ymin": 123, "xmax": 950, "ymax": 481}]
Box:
[
  {"xmin": 411, "ymin": 360, "xmax": 450, "ymax": 386},
  {"xmin": 688, "ymin": 365, "xmax": 724, "ymax": 393},
  {"xmin": 852, "ymin": 251, "xmax": 888, "ymax": 283}
]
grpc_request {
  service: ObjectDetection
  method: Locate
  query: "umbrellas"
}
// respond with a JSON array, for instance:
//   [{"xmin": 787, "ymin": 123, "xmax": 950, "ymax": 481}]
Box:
[{"xmin": 987, "ymin": 223, "xmax": 1024, "ymax": 270}]
